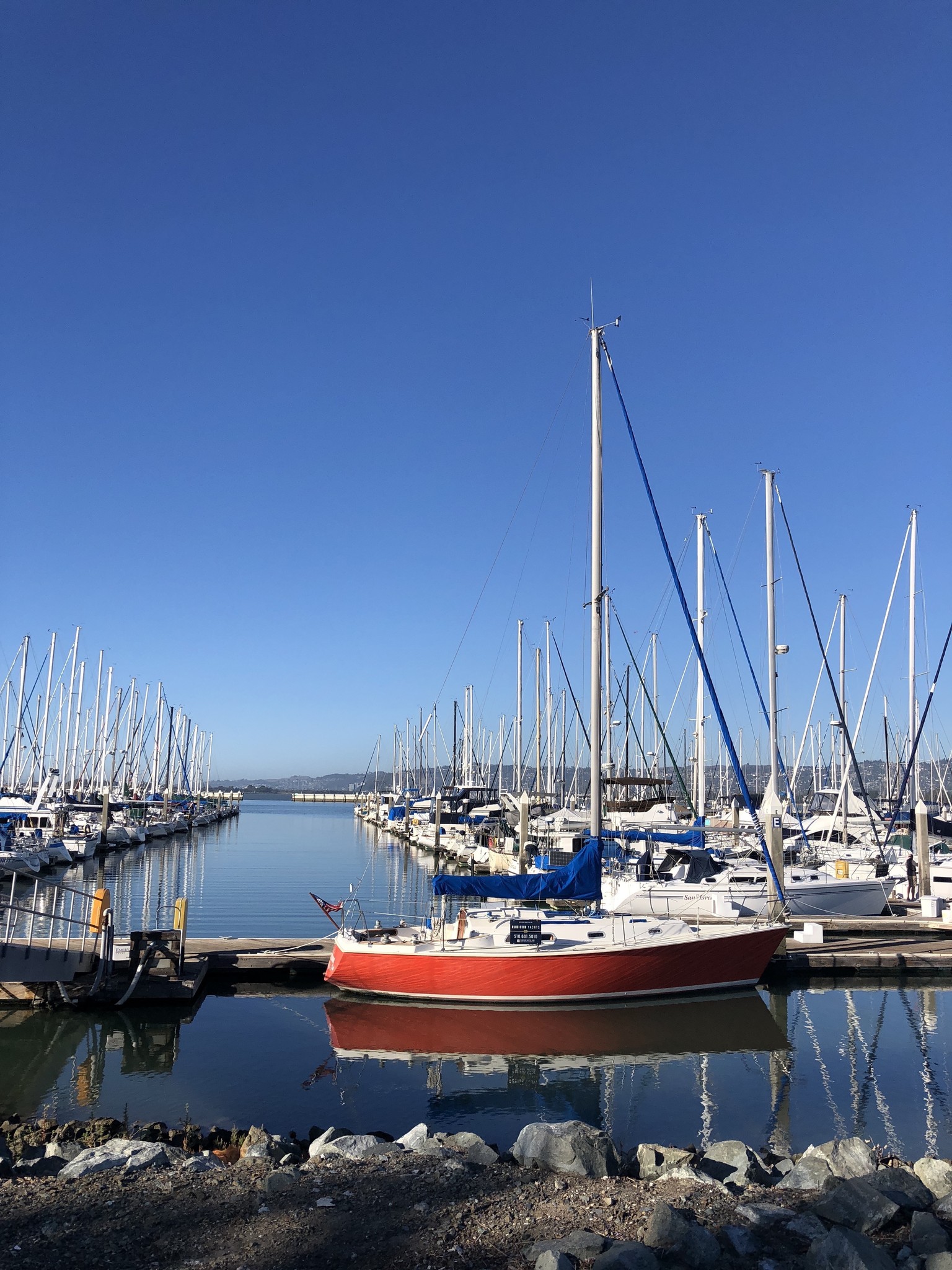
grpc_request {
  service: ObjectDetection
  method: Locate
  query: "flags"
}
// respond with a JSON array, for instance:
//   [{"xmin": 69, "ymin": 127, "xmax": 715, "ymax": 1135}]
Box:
[{"xmin": 312, "ymin": 893, "xmax": 343, "ymax": 914}]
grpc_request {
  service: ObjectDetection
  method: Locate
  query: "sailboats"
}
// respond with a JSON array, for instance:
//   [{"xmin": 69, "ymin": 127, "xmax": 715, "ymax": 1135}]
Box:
[
  {"xmin": 0, "ymin": 622, "xmax": 244, "ymax": 884},
  {"xmin": 320, "ymin": 985, "xmax": 795, "ymax": 1145},
  {"xmin": 307, "ymin": 314, "xmax": 800, "ymax": 1008},
  {"xmin": 351, "ymin": 459, "xmax": 951, "ymax": 929}
]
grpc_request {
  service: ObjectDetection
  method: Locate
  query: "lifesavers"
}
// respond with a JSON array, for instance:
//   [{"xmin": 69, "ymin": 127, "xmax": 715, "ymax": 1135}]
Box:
[
  {"xmin": 413, "ymin": 819, "xmax": 418, "ymax": 825},
  {"xmin": 83, "ymin": 824, "xmax": 91, "ymax": 835}
]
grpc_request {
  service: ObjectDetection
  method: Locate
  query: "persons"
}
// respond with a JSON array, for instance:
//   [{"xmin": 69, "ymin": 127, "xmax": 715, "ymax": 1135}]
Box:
[{"xmin": 905, "ymin": 854, "xmax": 918, "ymax": 901}]
